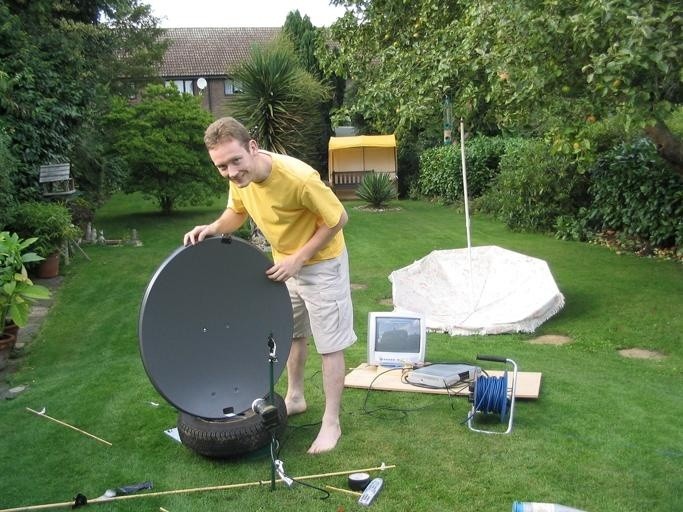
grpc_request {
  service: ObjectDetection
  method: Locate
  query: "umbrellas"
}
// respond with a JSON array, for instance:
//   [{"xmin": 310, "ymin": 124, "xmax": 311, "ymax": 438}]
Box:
[{"xmin": 388, "ymin": 115, "xmax": 565, "ymax": 337}]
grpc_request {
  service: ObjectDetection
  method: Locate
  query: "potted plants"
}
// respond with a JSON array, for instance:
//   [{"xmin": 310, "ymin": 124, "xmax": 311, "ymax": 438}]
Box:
[{"xmin": 5, "ymin": 201, "xmax": 85, "ymax": 278}]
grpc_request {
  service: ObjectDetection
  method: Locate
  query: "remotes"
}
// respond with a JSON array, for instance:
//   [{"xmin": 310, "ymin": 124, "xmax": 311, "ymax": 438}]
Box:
[{"xmin": 358, "ymin": 477, "xmax": 384, "ymax": 506}]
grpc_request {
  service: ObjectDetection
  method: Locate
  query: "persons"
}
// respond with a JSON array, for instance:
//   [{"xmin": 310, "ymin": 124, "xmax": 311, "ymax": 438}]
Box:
[{"xmin": 184, "ymin": 117, "xmax": 358, "ymax": 455}]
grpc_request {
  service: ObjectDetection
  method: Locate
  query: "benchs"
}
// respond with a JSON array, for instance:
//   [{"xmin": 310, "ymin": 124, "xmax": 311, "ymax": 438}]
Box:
[{"xmin": 332, "ymin": 169, "xmax": 374, "ymax": 195}]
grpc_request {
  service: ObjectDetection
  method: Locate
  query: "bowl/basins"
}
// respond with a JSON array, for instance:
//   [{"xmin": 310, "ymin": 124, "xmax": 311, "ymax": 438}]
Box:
[{"xmin": 346, "ymin": 473, "xmax": 371, "ymax": 491}]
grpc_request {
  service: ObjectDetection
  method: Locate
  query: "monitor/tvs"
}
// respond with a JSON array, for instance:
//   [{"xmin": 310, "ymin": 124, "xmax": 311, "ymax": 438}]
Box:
[{"xmin": 367, "ymin": 312, "xmax": 426, "ymax": 367}]
